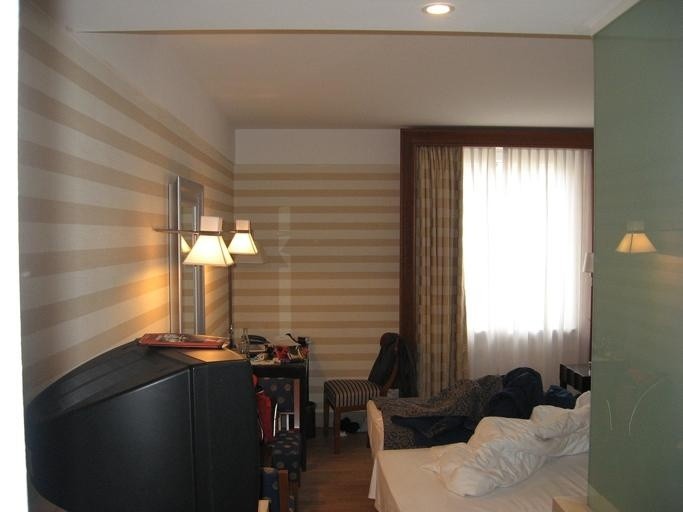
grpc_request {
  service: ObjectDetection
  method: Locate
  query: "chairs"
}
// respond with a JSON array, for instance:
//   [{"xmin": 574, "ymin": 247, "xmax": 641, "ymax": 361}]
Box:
[
  {"xmin": 322, "ymin": 330, "xmax": 401, "ymax": 457},
  {"xmin": 256, "ymin": 375, "xmax": 303, "ymax": 512}
]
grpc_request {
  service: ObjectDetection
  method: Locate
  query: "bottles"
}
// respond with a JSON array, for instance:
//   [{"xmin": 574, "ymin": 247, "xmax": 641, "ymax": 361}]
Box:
[{"xmin": 239, "ymin": 328, "xmax": 248, "ymax": 362}]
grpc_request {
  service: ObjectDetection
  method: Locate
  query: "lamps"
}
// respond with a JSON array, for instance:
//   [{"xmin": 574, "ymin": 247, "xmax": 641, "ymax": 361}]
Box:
[
  {"xmin": 152, "ymin": 213, "xmax": 237, "ymax": 270},
  {"xmin": 614, "ymin": 219, "xmax": 658, "ymax": 255},
  {"xmin": 582, "ymin": 250, "xmax": 593, "ymax": 369},
  {"xmin": 224, "ymin": 219, "xmax": 261, "ymax": 256}
]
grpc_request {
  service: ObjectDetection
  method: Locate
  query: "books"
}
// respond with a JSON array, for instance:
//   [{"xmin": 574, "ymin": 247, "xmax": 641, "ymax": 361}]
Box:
[{"xmin": 137, "ymin": 331, "xmax": 232, "ymax": 350}]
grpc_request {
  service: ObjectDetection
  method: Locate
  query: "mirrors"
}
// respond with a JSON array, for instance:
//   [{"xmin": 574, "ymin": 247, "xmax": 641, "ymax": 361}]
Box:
[{"xmin": 167, "ymin": 175, "xmax": 206, "ymax": 337}]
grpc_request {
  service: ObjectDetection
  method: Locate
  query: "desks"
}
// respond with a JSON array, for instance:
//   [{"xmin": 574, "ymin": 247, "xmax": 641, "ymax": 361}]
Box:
[{"xmin": 244, "ymin": 342, "xmax": 311, "ymax": 405}]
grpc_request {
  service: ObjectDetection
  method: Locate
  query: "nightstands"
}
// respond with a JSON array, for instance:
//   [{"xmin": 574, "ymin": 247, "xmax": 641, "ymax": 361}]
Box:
[{"xmin": 559, "ymin": 362, "xmax": 592, "ymax": 394}]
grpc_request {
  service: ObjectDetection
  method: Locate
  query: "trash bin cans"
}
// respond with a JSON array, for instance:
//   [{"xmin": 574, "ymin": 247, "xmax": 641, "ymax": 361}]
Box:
[{"xmin": 300, "ymin": 401, "xmax": 316, "ymax": 439}]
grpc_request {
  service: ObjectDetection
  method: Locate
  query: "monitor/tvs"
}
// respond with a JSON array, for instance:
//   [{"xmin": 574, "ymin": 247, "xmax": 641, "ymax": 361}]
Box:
[{"xmin": 25, "ymin": 337, "xmax": 261, "ymax": 512}]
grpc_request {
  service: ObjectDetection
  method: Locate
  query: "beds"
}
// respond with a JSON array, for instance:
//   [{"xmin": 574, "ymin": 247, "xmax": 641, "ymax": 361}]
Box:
[{"xmin": 363, "ymin": 391, "xmax": 590, "ymax": 512}]
